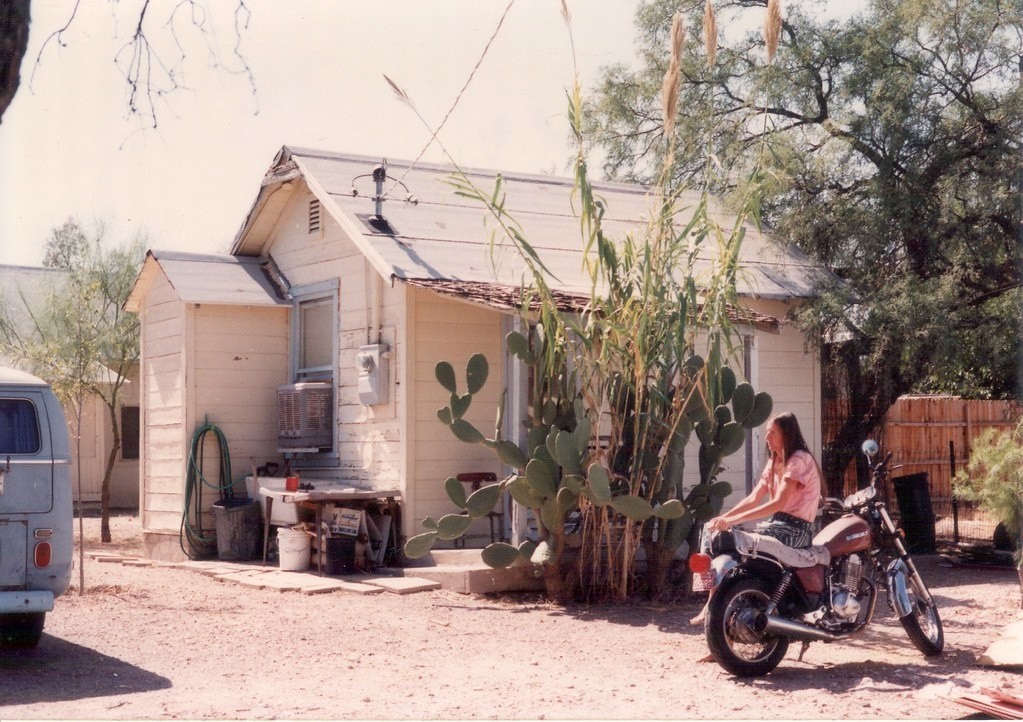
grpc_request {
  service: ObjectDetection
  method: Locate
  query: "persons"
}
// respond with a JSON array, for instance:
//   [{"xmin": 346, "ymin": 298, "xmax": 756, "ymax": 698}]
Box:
[{"xmin": 689, "ymin": 413, "xmax": 822, "ymax": 662}]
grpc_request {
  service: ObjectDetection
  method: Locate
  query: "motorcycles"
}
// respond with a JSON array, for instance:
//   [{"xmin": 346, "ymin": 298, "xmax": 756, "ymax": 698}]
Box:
[{"xmin": 689, "ymin": 439, "xmax": 944, "ymax": 677}]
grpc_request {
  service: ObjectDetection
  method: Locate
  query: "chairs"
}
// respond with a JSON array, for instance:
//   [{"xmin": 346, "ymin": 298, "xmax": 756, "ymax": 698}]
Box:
[{"xmin": 453, "ymin": 472, "xmax": 505, "ymax": 548}]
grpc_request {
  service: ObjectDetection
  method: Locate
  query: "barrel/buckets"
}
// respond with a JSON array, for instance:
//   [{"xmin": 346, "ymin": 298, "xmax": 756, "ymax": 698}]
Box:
[
  {"xmin": 277, "ymin": 533, "xmax": 312, "ymax": 570},
  {"xmin": 326, "ymin": 538, "xmax": 355, "ymax": 574},
  {"xmin": 901, "ymin": 515, "xmax": 936, "ymax": 556},
  {"xmin": 212, "ymin": 498, "xmax": 261, "ymax": 560},
  {"xmin": 892, "ymin": 473, "xmax": 932, "ymax": 519}
]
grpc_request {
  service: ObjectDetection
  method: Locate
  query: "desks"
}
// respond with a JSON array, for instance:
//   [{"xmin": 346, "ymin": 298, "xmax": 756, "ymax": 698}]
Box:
[{"xmin": 258, "ymin": 486, "xmax": 402, "ymax": 577}]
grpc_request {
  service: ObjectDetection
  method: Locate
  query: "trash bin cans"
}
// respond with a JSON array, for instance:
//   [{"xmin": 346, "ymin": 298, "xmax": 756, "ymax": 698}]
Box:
[{"xmin": 891, "ymin": 472, "xmax": 938, "ymax": 556}]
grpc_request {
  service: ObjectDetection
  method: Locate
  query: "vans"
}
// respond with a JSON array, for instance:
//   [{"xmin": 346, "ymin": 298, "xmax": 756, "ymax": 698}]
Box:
[{"xmin": 0, "ymin": 366, "xmax": 73, "ymax": 644}]
